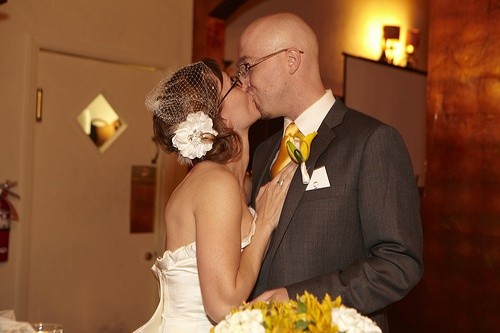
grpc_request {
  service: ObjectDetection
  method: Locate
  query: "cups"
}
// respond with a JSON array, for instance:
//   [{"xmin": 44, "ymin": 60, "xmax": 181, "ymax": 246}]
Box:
[{"xmin": 33, "ymin": 324, "xmax": 63, "ymax": 333}]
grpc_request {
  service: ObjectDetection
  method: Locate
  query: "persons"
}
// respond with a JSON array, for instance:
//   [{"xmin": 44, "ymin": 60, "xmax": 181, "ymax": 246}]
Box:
[
  {"xmin": 235, "ymin": 12, "xmax": 424, "ymax": 333},
  {"xmin": 129, "ymin": 57, "xmax": 298, "ymax": 333}
]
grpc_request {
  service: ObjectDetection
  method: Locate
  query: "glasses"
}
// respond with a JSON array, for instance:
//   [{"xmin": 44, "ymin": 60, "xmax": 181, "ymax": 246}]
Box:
[
  {"xmin": 217, "ymin": 75, "xmax": 243, "ymax": 112},
  {"xmin": 235, "ymin": 48, "xmax": 304, "ymax": 79}
]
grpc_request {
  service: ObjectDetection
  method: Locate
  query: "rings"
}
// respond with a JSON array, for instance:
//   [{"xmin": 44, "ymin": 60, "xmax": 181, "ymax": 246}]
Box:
[{"xmin": 278, "ymin": 180, "xmax": 284, "ymax": 186}]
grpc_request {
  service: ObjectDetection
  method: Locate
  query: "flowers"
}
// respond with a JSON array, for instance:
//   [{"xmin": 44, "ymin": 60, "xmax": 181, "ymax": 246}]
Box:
[
  {"xmin": 171, "ymin": 111, "xmax": 218, "ymax": 162},
  {"xmin": 285, "ymin": 130, "xmax": 320, "ymax": 185},
  {"xmin": 210, "ymin": 291, "xmax": 382, "ymax": 333}
]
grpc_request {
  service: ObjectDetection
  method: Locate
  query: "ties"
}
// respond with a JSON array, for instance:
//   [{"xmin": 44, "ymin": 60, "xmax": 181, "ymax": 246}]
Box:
[{"xmin": 270, "ymin": 122, "xmax": 299, "ymax": 179}]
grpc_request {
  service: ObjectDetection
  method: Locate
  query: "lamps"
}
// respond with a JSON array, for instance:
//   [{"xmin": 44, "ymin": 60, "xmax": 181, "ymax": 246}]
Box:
[{"xmin": 378, "ymin": 26, "xmax": 401, "ymax": 65}]
